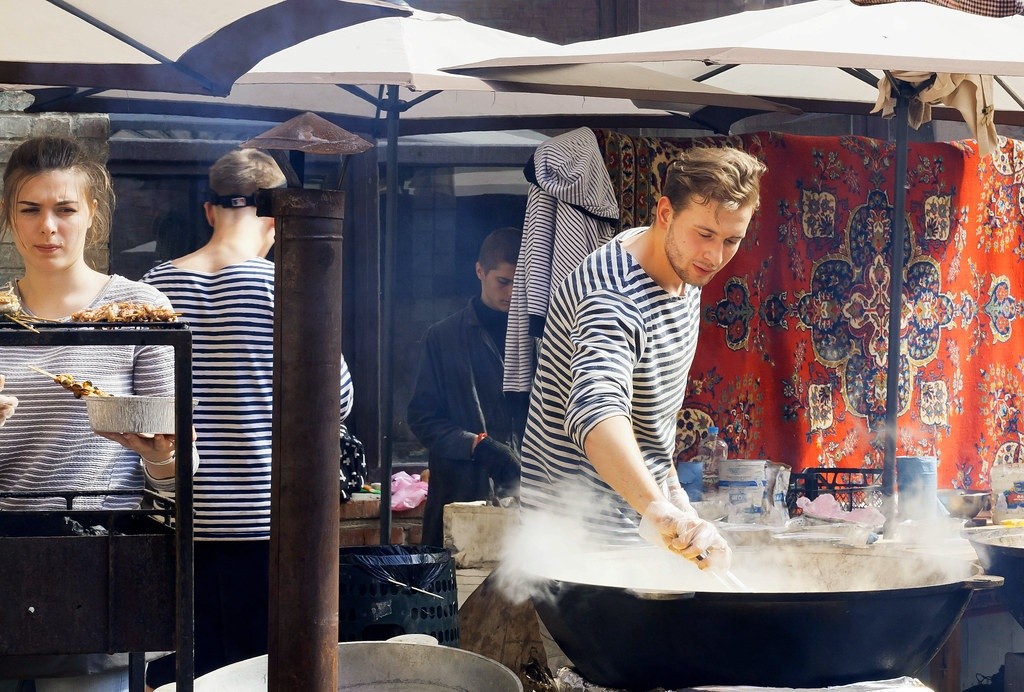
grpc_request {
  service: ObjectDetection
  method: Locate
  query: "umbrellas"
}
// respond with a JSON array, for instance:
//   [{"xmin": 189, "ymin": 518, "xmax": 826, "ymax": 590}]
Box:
[
  {"xmin": 336, "ymin": 128, "xmax": 561, "ymax": 180},
  {"xmin": 383, "ymin": 173, "xmax": 541, "ymax": 206},
  {"xmin": 0, "ymin": 0, "xmax": 419, "ymax": 106},
  {"xmin": 433, "ymin": 0, "xmax": 1024, "ymax": 129},
  {"xmin": 99, "ymin": 124, "xmax": 369, "ymax": 182},
  {"xmin": 50, "ymin": 0, "xmax": 720, "ymax": 168}
]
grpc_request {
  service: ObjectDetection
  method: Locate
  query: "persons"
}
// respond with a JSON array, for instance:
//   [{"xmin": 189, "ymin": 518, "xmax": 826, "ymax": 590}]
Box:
[
  {"xmin": 402, "ymin": 220, "xmax": 530, "ymax": 572},
  {"xmin": 517, "ymin": 141, "xmax": 764, "ymax": 587},
  {"xmin": 127, "ymin": 143, "xmax": 357, "ymax": 692},
  {"xmin": 135, "ymin": 178, "xmax": 210, "ymax": 266},
  {"xmin": 0, "ymin": 131, "xmax": 198, "ymax": 692}
]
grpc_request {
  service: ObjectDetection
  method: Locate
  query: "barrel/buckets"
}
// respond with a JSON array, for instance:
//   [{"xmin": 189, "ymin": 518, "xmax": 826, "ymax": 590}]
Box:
[
  {"xmin": 893, "ymin": 455, "xmax": 940, "ymax": 525},
  {"xmin": 717, "ymin": 458, "xmax": 766, "ymax": 521}
]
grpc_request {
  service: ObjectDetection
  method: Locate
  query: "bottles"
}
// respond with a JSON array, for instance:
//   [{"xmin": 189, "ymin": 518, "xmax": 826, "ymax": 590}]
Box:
[{"xmin": 697, "ymin": 427, "xmax": 729, "ymax": 497}]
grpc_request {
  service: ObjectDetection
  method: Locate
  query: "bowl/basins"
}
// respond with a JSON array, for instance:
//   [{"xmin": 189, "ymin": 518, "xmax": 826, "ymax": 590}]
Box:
[
  {"xmin": 82, "ymin": 393, "xmax": 199, "ymax": 435},
  {"xmin": 935, "ymin": 488, "xmax": 994, "ymax": 519}
]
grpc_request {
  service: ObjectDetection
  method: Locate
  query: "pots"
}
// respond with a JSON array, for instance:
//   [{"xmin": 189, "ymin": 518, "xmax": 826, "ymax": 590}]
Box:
[
  {"xmin": 968, "ymin": 526, "xmax": 1024, "ymax": 632},
  {"xmin": 517, "ymin": 545, "xmax": 1005, "ymax": 692}
]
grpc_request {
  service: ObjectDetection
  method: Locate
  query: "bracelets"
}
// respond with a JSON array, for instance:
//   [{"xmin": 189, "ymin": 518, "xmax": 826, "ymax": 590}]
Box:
[
  {"xmin": 139, "ymin": 451, "xmax": 179, "ymax": 466},
  {"xmin": 476, "ymin": 432, "xmax": 488, "ymax": 444}
]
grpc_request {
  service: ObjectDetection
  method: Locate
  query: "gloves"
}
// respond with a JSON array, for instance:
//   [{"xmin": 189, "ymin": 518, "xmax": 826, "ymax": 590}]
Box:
[{"xmin": 474, "ymin": 437, "xmax": 527, "ymax": 487}]
